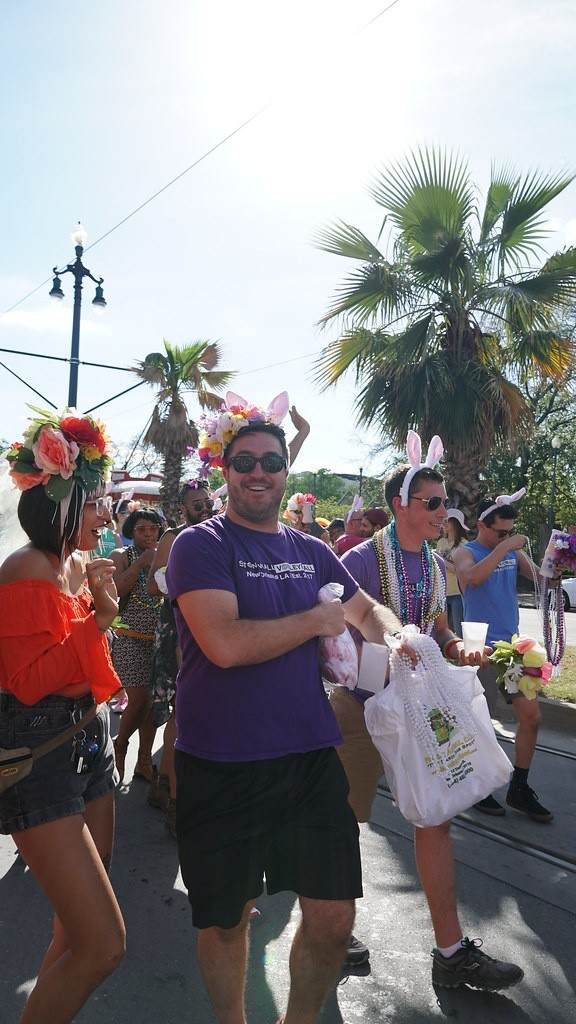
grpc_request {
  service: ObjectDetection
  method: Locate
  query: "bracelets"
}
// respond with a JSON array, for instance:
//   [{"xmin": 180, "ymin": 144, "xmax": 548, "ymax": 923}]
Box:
[{"xmin": 440, "ymin": 636, "xmax": 464, "ymax": 658}]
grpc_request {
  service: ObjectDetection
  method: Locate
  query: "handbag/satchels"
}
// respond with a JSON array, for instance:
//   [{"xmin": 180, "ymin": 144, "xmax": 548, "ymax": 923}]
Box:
[
  {"xmin": 362, "ymin": 640, "xmax": 516, "ymax": 829},
  {"xmin": 0, "ymin": 746, "xmax": 33, "ymax": 799}
]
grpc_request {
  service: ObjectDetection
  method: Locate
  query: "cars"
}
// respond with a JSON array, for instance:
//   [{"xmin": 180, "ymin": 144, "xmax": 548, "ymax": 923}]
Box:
[{"xmin": 547, "ymin": 576, "xmax": 575, "ymax": 613}]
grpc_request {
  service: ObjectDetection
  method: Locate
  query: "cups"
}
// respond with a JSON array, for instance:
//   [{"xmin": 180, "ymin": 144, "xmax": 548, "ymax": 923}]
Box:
[
  {"xmin": 461, "ymin": 622, "xmax": 488, "ymax": 656},
  {"xmin": 539, "ymin": 528, "xmax": 571, "ymax": 578}
]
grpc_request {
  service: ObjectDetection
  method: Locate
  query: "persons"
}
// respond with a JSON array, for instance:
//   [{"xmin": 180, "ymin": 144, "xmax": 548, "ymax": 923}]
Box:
[
  {"xmin": 1, "ymin": 409, "xmax": 128, "ymax": 1024},
  {"xmin": 109, "ymin": 480, "xmax": 567, "ymax": 821},
  {"xmin": 324, "ymin": 469, "xmax": 526, "ymax": 992},
  {"xmin": 166, "ymin": 421, "xmax": 419, "ymax": 1023}
]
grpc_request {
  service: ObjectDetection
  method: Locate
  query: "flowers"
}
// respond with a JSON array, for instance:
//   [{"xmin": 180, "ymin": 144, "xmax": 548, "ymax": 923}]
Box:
[
  {"xmin": 182, "ymin": 405, "xmax": 272, "ymax": 489},
  {"xmin": 6, "ymin": 401, "xmax": 116, "ymax": 499},
  {"xmin": 449, "ymin": 634, "xmax": 553, "ymax": 700},
  {"xmin": 128, "ymin": 500, "xmax": 140, "ymax": 513},
  {"xmin": 282, "ymin": 492, "xmax": 316, "ymax": 524},
  {"xmin": 546, "ymin": 531, "xmax": 576, "ymax": 574}
]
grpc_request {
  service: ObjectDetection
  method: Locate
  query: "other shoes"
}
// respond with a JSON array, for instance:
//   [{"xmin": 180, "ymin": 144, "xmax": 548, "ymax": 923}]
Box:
[
  {"xmin": 112, "ymin": 696, "xmax": 129, "ymax": 713},
  {"xmin": 250, "ymin": 907, "xmax": 261, "ymax": 919}
]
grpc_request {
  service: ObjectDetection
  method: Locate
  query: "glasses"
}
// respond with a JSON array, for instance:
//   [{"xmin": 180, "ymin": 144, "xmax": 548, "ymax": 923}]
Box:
[
  {"xmin": 397, "ymin": 494, "xmax": 451, "ymax": 511},
  {"xmin": 484, "ymin": 522, "xmax": 517, "ymax": 539},
  {"xmin": 182, "ymin": 499, "xmax": 216, "ymax": 513},
  {"xmin": 227, "ymin": 451, "xmax": 287, "ymax": 475},
  {"xmin": 85, "ymin": 495, "xmax": 113, "ymax": 517},
  {"xmin": 135, "ymin": 524, "xmax": 160, "ymax": 535}
]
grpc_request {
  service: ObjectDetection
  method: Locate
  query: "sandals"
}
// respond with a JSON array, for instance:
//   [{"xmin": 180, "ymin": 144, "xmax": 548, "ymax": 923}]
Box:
[
  {"xmin": 114, "ymin": 738, "xmax": 129, "ymax": 782},
  {"xmin": 133, "ymin": 751, "xmax": 157, "ymax": 783}
]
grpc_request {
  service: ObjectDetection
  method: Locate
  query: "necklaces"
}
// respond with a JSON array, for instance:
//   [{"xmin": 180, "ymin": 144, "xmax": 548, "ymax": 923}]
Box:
[
  {"xmin": 130, "ymin": 545, "xmax": 165, "ymax": 609},
  {"xmin": 368, "ymin": 519, "xmax": 446, "ymax": 662},
  {"xmin": 520, "ymin": 535, "xmax": 567, "ymax": 665}
]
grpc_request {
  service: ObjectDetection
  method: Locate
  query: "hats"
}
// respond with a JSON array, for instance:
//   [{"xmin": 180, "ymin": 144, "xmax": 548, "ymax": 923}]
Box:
[
  {"xmin": 443, "ymin": 507, "xmax": 470, "ymax": 533},
  {"xmin": 324, "ymin": 519, "xmax": 345, "ymax": 530},
  {"xmin": 363, "ymin": 508, "xmax": 387, "ymax": 527}
]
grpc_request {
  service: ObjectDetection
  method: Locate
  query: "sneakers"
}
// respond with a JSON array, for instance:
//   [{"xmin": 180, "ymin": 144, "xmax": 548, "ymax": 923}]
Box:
[
  {"xmin": 506, "ymin": 779, "xmax": 553, "ymax": 821},
  {"xmin": 164, "ymin": 799, "xmax": 177, "ymax": 840},
  {"xmin": 342, "ymin": 936, "xmax": 369, "ymax": 966},
  {"xmin": 473, "ymin": 795, "xmax": 506, "ymax": 816},
  {"xmin": 148, "ymin": 771, "xmax": 171, "ymax": 812},
  {"xmin": 431, "ymin": 937, "xmax": 524, "ymax": 992}
]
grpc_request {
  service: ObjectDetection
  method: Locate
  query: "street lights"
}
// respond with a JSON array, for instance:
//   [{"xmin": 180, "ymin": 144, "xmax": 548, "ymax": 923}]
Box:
[{"xmin": 46, "ymin": 240, "xmax": 107, "ymax": 412}]
